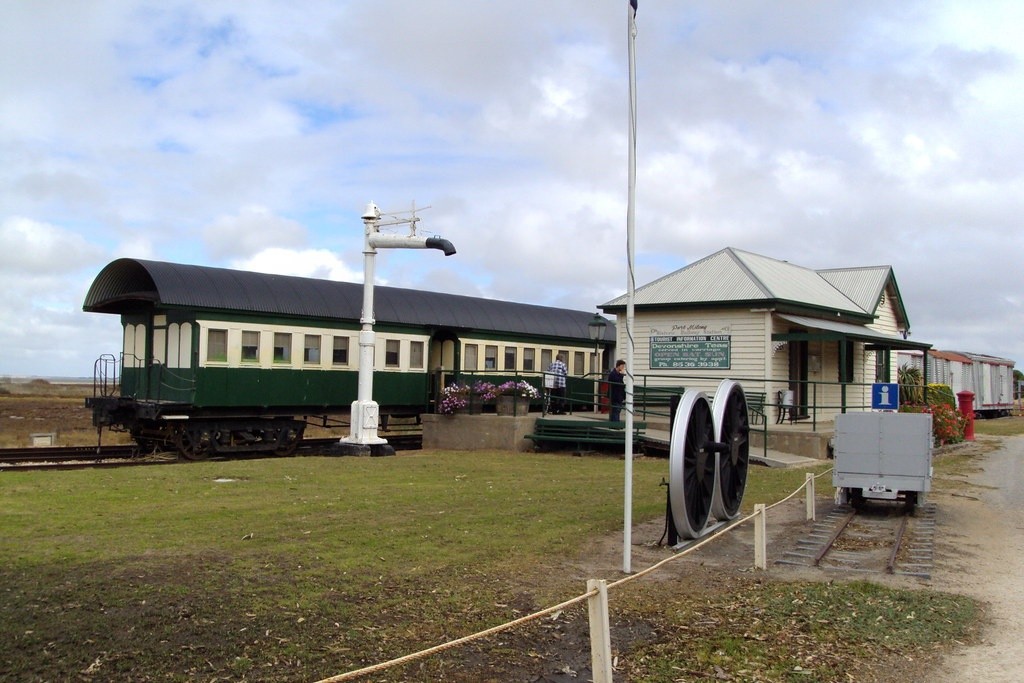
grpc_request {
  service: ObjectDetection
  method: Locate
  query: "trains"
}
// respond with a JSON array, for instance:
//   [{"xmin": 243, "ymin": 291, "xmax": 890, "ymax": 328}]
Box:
[{"xmin": 82, "ymin": 257, "xmax": 615, "ymax": 461}]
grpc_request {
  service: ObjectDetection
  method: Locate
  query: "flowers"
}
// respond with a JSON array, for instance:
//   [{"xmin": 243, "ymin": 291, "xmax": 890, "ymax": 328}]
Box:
[
  {"xmin": 480, "ymin": 380, "xmax": 541, "ymax": 403},
  {"xmin": 438, "ymin": 380, "xmax": 495, "ymax": 414}
]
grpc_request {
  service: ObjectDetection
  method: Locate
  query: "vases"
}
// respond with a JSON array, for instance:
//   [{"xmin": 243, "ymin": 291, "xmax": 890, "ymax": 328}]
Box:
[
  {"xmin": 452, "ymin": 392, "xmax": 483, "ymax": 414},
  {"xmin": 496, "ymin": 395, "xmax": 530, "ymax": 416}
]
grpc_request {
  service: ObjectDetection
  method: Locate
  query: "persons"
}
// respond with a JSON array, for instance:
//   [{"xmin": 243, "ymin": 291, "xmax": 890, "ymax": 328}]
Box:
[
  {"xmin": 547, "ymin": 354, "xmax": 568, "ymax": 415},
  {"xmin": 609, "ymin": 360, "xmax": 626, "ymax": 430}
]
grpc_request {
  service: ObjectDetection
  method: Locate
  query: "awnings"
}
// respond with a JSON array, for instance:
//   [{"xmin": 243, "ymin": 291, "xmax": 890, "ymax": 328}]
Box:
[{"xmin": 774, "ymin": 314, "xmax": 934, "ymax": 347}]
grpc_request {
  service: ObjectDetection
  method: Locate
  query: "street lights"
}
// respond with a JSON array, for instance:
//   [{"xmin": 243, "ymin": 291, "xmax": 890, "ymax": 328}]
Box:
[{"xmin": 588, "ymin": 310, "xmax": 608, "ymax": 413}]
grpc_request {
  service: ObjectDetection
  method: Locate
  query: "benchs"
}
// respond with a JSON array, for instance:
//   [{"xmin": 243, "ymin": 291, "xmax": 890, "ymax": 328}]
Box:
[
  {"xmin": 523, "ymin": 419, "xmax": 648, "ymax": 461},
  {"xmin": 622, "ymin": 385, "xmax": 767, "ymax": 425}
]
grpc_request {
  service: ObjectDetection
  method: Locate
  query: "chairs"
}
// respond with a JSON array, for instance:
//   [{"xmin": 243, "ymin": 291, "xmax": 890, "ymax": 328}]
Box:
[{"xmin": 776, "ymin": 391, "xmax": 798, "ymax": 425}]
